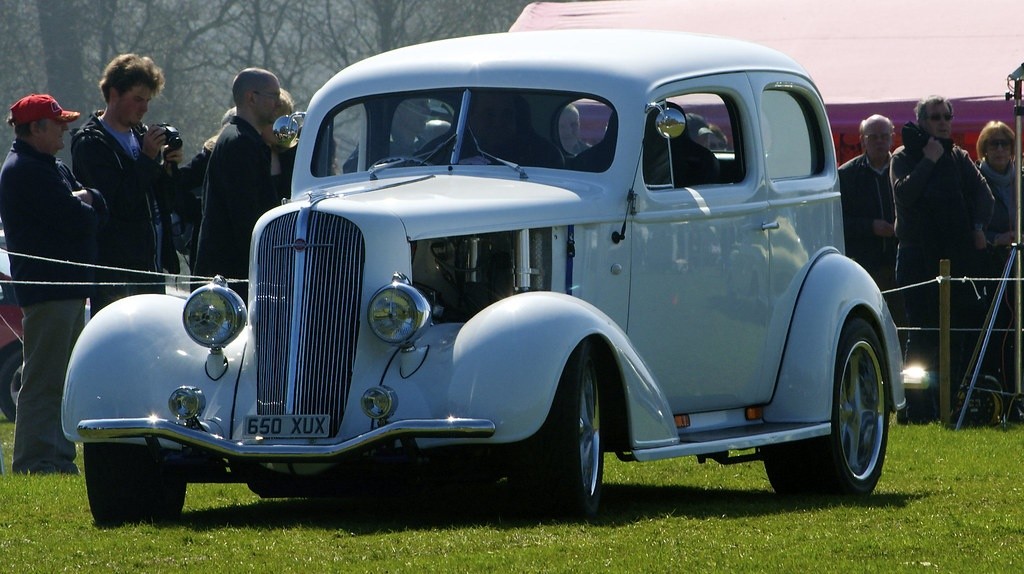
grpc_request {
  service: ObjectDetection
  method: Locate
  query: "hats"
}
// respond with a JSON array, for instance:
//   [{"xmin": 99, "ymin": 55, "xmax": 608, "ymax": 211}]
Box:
[
  {"xmin": 685, "ymin": 113, "xmax": 713, "ymax": 138},
  {"xmin": 9, "ymin": 94, "xmax": 80, "ymax": 125}
]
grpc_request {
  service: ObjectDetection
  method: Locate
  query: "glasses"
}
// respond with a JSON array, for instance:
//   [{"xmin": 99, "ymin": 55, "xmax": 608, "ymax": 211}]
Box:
[
  {"xmin": 985, "ymin": 140, "xmax": 1012, "ymax": 151},
  {"xmin": 864, "ymin": 133, "xmax": 893, "ymax": 142},
  {"xmin": 709, "ymin": 141, "xmax": 724, "ymax": 149},
  {"xmin": 923, "ymin": 114, "xmax": 955, "ymax": 122}
]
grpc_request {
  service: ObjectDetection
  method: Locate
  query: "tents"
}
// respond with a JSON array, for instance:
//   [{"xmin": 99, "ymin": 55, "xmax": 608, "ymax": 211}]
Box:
[{"xmin": 508, "ymin": 0, "xmax": 1024, "ymax": 167}]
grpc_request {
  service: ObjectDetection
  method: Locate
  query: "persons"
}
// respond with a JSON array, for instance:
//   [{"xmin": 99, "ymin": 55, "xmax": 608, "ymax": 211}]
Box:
[
  {"xmin": 559, "ymin": 104, "xmax": 592, "ymax": 154},
  {"xmin": 686, "ymin": 114, "xmax": 727, "ymax": 151},
  {"xmin": 343, "ymin": 99, "xmax": 451, "ymax": 173},
  {"xmin": 0, "ymin": 54, "xmax": 301, "ymax": 474},
  {"xmin": 838, "ymin": 96, "xmax": 1024, "ymax": 423}
]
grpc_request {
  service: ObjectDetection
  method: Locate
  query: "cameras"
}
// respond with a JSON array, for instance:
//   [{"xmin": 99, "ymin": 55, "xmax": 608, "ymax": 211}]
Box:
[{"xmin": 157, "ymin": 123, "xmax": 182, "ymax": 149}]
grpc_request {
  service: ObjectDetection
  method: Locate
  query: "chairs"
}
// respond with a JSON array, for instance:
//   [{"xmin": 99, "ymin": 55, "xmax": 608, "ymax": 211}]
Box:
[
  {"xmin": 563, "ymin": 101, "xmax": 726, "ymax": 192},
  {"xmin": 410, "ymin": 88, "xmax": 563, "ymax": 169}
]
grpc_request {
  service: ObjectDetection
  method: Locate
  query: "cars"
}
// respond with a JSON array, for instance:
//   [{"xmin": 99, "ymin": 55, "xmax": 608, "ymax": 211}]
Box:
[{"xmin": 61, "ymin": 33, "xmax": 908, "ymax": 524}]
